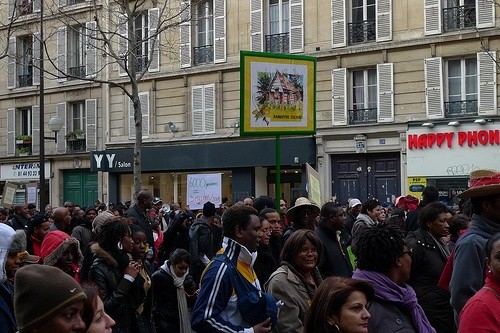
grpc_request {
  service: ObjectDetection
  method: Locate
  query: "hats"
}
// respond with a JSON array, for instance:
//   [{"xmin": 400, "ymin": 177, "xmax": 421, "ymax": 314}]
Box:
[
  {"xmin": 108, "ymin": 202, "xmax": 115, "ymax": 206},
  {"xmin": 28, "ymin": 203, "xmax": 37, "ymax": 210},
  {"xmin": 455, "ymin": 170, "xmax": 500, "ymax": 201},
  {"xmin": 202, "ymin": 201, "xmax": 215, "ymax": 217},
  {"xmin": 152, "ymin": 197, "xmax": 164, "ymax": 205},
  {"xmin": 286, "ymin": 197, "xmax": 320, "ymax": 222},
  {"xmin": 83, "ymin": 205, "xmax": 98, "ymax": 217},
  {"xmin": 349, "ymin": 198, "xmax": 362, "ymax": 208},
  {"xmin": 91, "ymin": 210, "xmax": 117, "ymax": 236},
  {"xmin": 14, "ymin": 263, "xmax": 87, "ymax": 331}
]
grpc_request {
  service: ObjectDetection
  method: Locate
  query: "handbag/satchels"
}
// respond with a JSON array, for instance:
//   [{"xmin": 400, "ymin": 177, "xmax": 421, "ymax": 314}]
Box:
[{"xmin": 235, "ymin": 284, "xmax": 279, "ymax": 332}]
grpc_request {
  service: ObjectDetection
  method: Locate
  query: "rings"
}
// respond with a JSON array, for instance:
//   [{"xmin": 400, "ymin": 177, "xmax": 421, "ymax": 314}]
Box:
[
  {"xmin": 135, "ymin": 266, "xmax": 137, "ymax": 267},
  {"xmin": 128, "ymin": 263, "xmax": 131, "ymax": 264},
  {"xmin": 132, "ymin": 264, "xmax": 134, "ymax": 266}
]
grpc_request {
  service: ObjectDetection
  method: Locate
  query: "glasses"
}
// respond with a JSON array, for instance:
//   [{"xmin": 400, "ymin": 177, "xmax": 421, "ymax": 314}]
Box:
[{"xmin": 401, "ymin": 247, "xmax": 414, "ymax": 255}]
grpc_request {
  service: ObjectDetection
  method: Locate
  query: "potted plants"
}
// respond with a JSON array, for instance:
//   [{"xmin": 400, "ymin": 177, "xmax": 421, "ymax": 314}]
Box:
[
  {"xmin": 15, "ymin": 146, "xmax": 31, "ymax": 156},
  {"xmin": 66, "ymin": 129, "xmax": 86, "ymax": 141},
  {"xmin": 15, "ymin": 135, "xmax": 32, "ymax": 144}
]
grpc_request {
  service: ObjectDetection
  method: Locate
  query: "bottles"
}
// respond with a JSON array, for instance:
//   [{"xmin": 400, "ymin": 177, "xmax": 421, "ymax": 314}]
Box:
[{"xmin": 144, "ymin": 239, "xmax": 154, "ymax": 259}]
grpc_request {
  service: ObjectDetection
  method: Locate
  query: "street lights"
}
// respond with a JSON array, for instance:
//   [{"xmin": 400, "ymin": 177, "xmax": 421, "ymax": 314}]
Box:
[{"xmin": 40, "ymin": 116, "xmax": 62, "ymax": 212}]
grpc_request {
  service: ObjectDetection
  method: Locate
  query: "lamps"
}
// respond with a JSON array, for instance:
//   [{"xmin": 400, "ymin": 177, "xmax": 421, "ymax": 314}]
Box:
[
  {"xmin": 474, "ymin": 119, "xmax": 487, "ymax": 125},
  {"xmin": 448, "ymin": 121, "xmax": 460, "ymax": 127},
  {"xmin": 422, "ymin": 123, "xmax": 434, "ymax": 128}
]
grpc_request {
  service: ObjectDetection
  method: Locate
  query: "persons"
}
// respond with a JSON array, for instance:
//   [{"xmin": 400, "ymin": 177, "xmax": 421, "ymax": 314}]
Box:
[
  {"xmin": 145, "ymin": 246, "xmax": 197, "ymax": 333},
  {"xmin": 87, "ymin": 220, "xmax": 143, "ymax": 333},
  {"xmin": 190, "ymin": 203, "xmax": 281, "ymax": 333},
  {"xmin": 350, "ymin": 221, "xmax": 436, "ymax": 333},
  {"xmin": 0, "ymin": 169, "xmax": 500, "ymax": 333}
]
